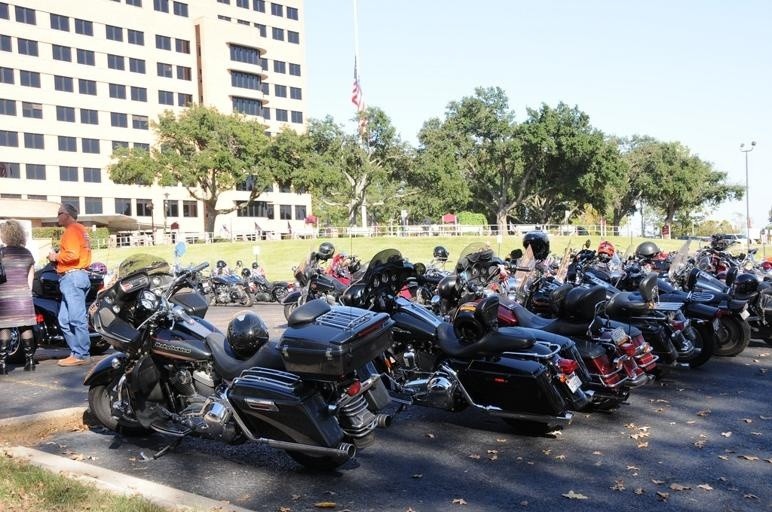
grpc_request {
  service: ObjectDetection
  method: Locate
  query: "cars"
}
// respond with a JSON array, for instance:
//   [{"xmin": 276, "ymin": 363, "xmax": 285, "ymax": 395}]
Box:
[
  {"xmin": 515, "ymin": 224, "xmax": 540, "ymax": 238},
  {"xmin": 558, "ymin": 223, "xmax": 590, "ymax": 236}
]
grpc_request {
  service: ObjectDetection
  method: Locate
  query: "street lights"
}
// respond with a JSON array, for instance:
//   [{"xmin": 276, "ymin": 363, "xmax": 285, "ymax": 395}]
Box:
[
  {"xmin": 161, "ymin": 190, "xmax": 170, "ymax": 234},
  {"xmin": 740, "ymin": 139, "xmax": 758, "ymax": 250},
  {"xmin": 144, "ymin": 200, "xmax": 155, "ymax": 246}
]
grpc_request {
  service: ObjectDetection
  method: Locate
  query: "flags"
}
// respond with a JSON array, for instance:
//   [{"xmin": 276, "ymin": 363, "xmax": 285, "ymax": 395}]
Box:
[{"xmin": 352, "ymin": 56, "xmax": 362, "ymax": 107}]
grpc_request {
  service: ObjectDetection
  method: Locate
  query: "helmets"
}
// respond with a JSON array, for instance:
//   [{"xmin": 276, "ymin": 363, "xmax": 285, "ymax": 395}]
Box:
[
  {"xmin": 522, "ymin": 230, "xmax": 550, "ymax": 261},
  {"xmin": 318, "ymin": 242, "xmax": 335, "ymax": 259},
  {"xmin": 635, "ymin": 241, "xmax": 660, "ymax": 259},
  {"xmin": 433, "ymin": 246, "xmax": 449, "ymax": 261},
  {"xmin": 597, "ymin": 241, "xmax": 614, "ymax": 257},
  {"xmin": 452, "ymin": 302, "xmax": 485, "ymax": 346},
  {"xmin": 226, "ymin": 309, "xmax": 270, "ymax": 360},
  {"xmin": 216, "ymin": 260, "xmax": 258, "ymax": 277},
  {"xmin": 732, "ymin": 274, "xmax": 759, "ymax": 295}
]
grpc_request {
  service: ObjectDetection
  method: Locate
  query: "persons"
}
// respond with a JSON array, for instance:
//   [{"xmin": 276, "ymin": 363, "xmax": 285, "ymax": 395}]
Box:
[
  {"xmin": 0, "ymin": 219, "xmax": 36, "ymax": 375},
  {"xmin": 47, "ymin": 202, "xmax": 91, "ymax": 367}
]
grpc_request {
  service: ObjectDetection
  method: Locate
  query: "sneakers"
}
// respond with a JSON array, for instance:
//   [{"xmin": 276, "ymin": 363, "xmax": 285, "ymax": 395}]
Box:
[{"xmin": 57, "ymin": 354, "xmax": 91, "ymax": 368}]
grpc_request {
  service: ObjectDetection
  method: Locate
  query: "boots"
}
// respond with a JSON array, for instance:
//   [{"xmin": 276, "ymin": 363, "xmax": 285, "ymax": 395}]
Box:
[
  {"xmin": 22, "ymin": 337, "xmax": 36, "ymax": 372},
  {"xmin": 0, "ymin": 339, "xmax": 11, "ymax": 376}
]
